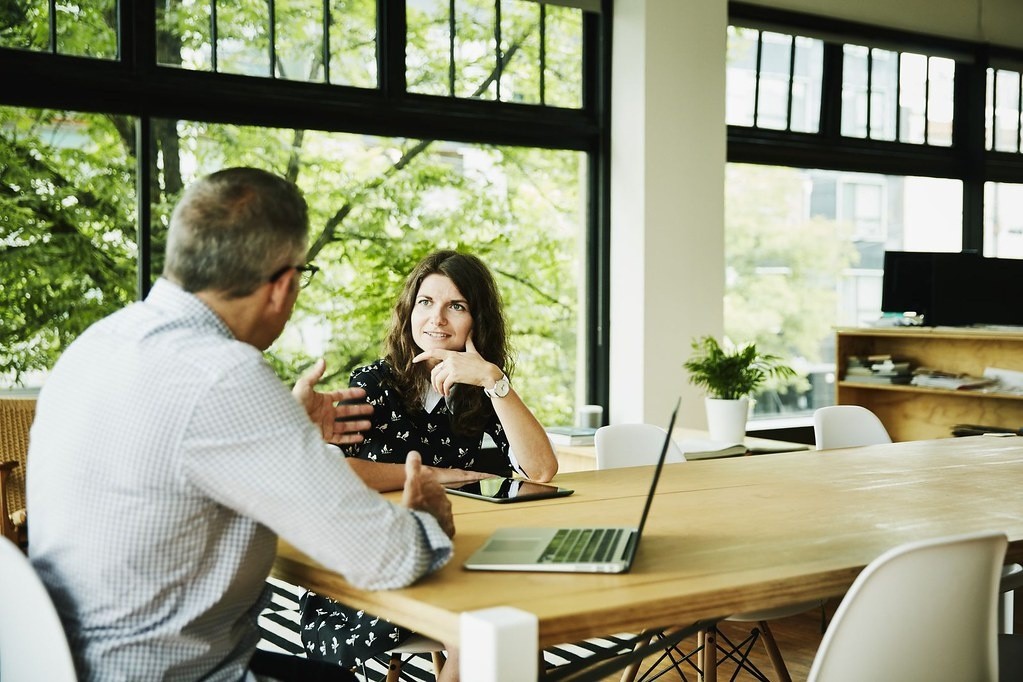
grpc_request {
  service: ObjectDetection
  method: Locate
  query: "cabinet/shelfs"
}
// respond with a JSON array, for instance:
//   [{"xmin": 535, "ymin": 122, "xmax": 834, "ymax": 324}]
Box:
[{"xmin": 835, "ymin": 327, "xmax": 1023, "ymax": 445}]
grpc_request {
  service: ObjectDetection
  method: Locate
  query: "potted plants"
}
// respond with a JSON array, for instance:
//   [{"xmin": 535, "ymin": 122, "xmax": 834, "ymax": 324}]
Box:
[{"xmin": 683, "ymin": 337, "xmax": 798, "ymax": 440}]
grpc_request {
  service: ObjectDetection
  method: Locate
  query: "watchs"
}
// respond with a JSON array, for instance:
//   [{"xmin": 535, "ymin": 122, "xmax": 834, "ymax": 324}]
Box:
[{"xmin": 484, "ymin": 373, "xmax": 510, "ymax": 399}]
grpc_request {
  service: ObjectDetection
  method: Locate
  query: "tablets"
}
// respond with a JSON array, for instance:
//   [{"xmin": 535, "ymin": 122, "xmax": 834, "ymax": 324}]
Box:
[{"xmin": 441, "ymin": 476, "xmax": 576, "ymax": 503}]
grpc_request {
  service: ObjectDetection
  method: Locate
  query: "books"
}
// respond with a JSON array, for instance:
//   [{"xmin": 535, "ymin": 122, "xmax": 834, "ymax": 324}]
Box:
[
  {"xmin": 676, "ymin": 439, "xmax": 809, "ymax": 461},
  {"xmin": 544, "ymin": 426, "xmax": 599, "ymax": 447}
]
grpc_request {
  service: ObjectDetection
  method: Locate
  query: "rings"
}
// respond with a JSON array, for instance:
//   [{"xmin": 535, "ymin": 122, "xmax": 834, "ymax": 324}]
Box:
[{"xmin": 439, "ymin": 365, "xmax": 442, "ymax": 370}]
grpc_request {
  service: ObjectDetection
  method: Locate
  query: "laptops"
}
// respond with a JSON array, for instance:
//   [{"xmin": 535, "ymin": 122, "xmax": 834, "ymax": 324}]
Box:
[{"xmin": 463, "ymin": 396, "xmax": 683, "ymax": 574}]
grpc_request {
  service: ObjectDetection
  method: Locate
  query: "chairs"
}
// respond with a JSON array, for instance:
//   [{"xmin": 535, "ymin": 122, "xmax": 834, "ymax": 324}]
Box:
[
  {"xmin": 596, "ymin": 425, "xmax": 825, "ymax": 682},
  {"xmin": 0, "ymin": 399, "xmax": 78, "ymax": 682},
  {"xmin": 814, "ymin": 404, "xmax": 894, "ymax": 451},
  {"xmin": 807, "ymin": 529, "xmax": 1008, "ymax": 682}
]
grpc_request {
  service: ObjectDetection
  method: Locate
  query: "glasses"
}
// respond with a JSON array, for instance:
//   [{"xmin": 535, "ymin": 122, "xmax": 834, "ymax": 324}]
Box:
[{"xmin": 269, "ymin": 264, "xmax": 320, "ymax": 288}]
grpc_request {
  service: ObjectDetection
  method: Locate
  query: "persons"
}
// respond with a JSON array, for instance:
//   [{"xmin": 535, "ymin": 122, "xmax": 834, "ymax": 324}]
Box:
[
  {"xmin": 299, "ymin": 249, "xmax": 558, "ymax": 682},
  {"xmin": 25, "ymin": 167, "xmax": 455, "ymax": 682}
]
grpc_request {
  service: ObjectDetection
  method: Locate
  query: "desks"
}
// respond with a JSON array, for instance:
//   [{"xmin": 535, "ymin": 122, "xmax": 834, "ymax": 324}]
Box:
[
  {"xmin": 271, "ymin": 433, "xmax": 1023, "ymax": 682},
  {"xmin": 547, "ymin": 427, "xmax": 812, "ymax": 478}
]
